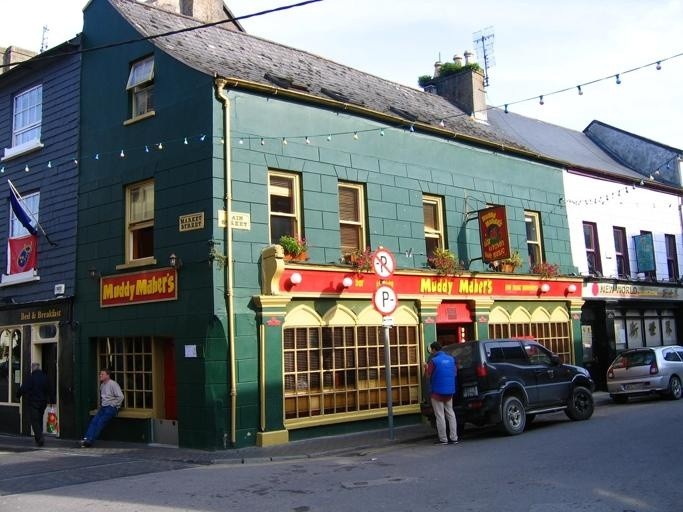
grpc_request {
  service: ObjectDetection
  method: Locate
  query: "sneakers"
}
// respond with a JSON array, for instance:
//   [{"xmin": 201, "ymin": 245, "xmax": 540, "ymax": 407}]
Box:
[{"xmin": 433, "ymin": 439, "xmax": 460, "ymax": 446}]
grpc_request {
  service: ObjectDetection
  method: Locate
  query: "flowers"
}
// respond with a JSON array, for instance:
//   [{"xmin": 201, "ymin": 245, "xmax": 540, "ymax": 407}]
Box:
[
  {"xmin": 279, "ymin": 235, "xmax": 308, "ymax": 256},
  {"xmin": 354, "ymin": 248, "xmax": 373, "ymax": 271},
  {"xmin": 533, "ymin": 263, "xmax": 560, "ymax": 279}
]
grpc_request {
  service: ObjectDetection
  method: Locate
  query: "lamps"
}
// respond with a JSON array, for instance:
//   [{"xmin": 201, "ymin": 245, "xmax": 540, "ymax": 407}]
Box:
[
  {"xmin": 342, "ymin": 277, "xmax": 352, "ymax": 289},
  {"xmin": 567, "ymin": 285, "xmax": 577, "ymax": 293},
  {"xmin": 288, "ymin": 273, "xmax": 302, "ymax": 288},
  {"xmin": 540, "ymin": 283, "xmax": 550, "ymax": 293},
  {"xmin": 88, "ymin": 265, "xmax": 101, "ymax": 281},
  {"xmin": 169, "ymin": 253, "xmax": 183, "ymax": 270}
]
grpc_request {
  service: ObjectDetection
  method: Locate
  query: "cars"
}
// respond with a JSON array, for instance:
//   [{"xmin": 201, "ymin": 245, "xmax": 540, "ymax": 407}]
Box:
[{"xmin": 606, "ymin": 345, "xmax": 683, "ymax": 404}]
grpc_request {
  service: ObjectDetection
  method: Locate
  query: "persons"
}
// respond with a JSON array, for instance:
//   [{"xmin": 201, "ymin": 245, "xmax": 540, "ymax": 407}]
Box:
[
  {"xmin": 13, "ymin": 361, "xmax": 56, "ymax": 448},
  {"xmin": 76, "ymin": 367, "xmax": 126, "ymax": 448},
  {"xmin": 423, "ymin": 341, "xmax": 460, "ymax": 446}
]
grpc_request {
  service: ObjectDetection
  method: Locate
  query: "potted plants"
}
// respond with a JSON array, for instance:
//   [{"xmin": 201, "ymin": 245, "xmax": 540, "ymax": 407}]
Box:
[
  {"xmin": 495, "ymin": 251, "xmax": 522, "ymax": 273},
  {"xmin": 428, "ymin": 247, "xmax": 464, "ymax": 278}
]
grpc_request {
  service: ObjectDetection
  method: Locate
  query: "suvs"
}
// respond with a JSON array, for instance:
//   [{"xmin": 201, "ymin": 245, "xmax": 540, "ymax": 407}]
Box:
[{"xmin": 420, "ymin": 338, "xmax": 595, "ymax": 436}]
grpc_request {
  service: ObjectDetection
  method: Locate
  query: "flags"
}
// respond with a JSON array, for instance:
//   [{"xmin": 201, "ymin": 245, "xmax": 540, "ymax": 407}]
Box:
[
  {"xmin": 6, "ymin": 236, "xmax": 38, "ymax": 276},
  {"xmin": 6, "ymin": 184, "xmax": 38, "ymax": 237}
]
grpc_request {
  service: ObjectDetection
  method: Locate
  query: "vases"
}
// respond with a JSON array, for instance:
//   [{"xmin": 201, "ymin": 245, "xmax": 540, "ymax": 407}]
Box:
[
  {"xmin": 345, "ymin": 255, "xmax": 358, "ymax": 264},
  {"xmin": 282, "ymin": 251, "xmax": 306, "ymax": 260}
]
